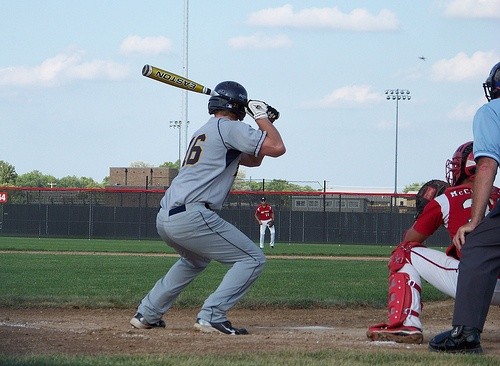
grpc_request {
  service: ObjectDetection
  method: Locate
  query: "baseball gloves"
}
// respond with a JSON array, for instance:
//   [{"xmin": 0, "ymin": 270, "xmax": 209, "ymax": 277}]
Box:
[
  {"xmin": 267, "ymin": 221, "xmax": 274, "ymax": 227},
  {"xmin": 414, "ymin": 180, "xmax": 450, "ymax": 217}
]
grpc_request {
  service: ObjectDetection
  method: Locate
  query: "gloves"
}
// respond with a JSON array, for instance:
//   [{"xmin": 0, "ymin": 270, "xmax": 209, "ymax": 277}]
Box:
[{"xmin": 247, "ymin": 100, "xmax": 268, "ymax": 120}]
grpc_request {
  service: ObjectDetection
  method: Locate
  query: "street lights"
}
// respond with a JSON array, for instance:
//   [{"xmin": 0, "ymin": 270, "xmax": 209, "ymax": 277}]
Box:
[
  {"xmin": 383, "ymin": 88, "xmax": 412, "ymax": 206},
  {"xmin": 169, "ymin": 120, "xmax": 190, "ymax": 169}
]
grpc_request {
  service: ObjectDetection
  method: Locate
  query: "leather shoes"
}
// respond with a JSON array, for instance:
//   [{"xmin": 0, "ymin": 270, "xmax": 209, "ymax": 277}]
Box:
[{"xmin": 430, "ymin": 326, "xmax": 482, "ymax": 354}]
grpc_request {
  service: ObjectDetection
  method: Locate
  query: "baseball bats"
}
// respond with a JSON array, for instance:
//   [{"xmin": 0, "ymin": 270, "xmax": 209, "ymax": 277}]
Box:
[{"xmin": 142, "ymin": 64, "xmax": 281, "ymax": 120}]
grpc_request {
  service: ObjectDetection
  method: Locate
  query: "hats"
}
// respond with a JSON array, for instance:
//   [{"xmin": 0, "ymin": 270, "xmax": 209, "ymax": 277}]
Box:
[{"xmin": 261, "ymin": 197, "xmax": 266, "ymax": 200}]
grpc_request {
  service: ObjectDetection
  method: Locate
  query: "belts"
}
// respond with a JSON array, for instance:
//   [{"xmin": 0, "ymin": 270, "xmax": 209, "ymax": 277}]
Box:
[{"xmin": 158, "ymin": 203, "xmax": 210, "ymax": 216}]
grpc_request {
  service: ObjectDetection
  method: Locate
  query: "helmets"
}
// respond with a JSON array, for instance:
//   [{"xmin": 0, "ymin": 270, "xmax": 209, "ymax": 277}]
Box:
[
  {"xmin": 446, "ymin": 141, "xmax": 477, "ymax": 186},
  {"xmin": 482, "ymin": 62, "xmax": 500, "ymax": 102},
  {"xmin": 208, "ymin": 81, "xmax": 246, "ymax": 121}
]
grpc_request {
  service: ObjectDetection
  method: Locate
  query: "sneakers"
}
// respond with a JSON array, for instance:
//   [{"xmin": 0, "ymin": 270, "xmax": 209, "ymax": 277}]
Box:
[
  {"xmin": 366, "ymin": 322, "xmax": 424, "ymax": 344},
  {"xmin": 194, "ymin": 318, "xmax": 248, "ymax": 335},
  {"xmin": 130, "ymin": 313, "xmax": 166, "ymax": 330}
]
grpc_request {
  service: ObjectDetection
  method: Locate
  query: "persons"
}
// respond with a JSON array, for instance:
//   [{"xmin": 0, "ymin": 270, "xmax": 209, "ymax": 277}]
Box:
[
  {"xmin": 366, "ymin": 142, "xmax": 500, "ymax": 343},
  {"xmin": 132, "ymin": 81, "xmax": 286, "ymax": 335},
  {"xmin": 428, "ymin": 62, "xmax": 500, "ymax": 351},
  {"xmin": 254, "ymin": 197, "xmax": 276, "ymax": 249}
]
